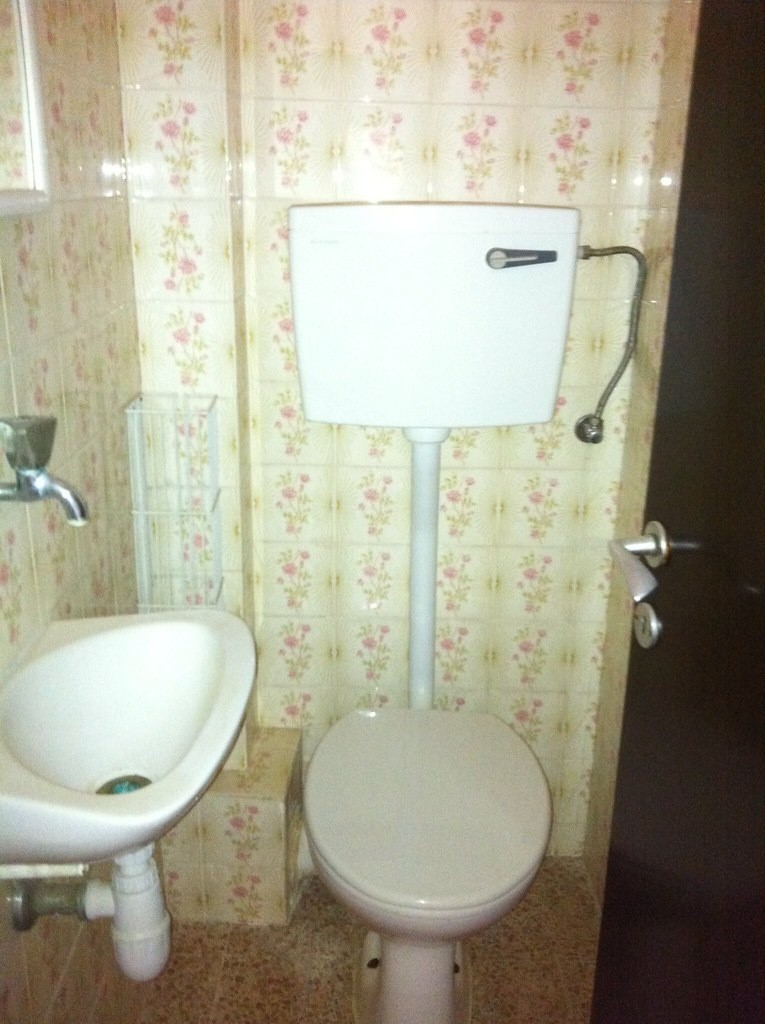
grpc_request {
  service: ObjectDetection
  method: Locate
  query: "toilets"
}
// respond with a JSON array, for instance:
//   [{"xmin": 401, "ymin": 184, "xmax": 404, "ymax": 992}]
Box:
[{"xmin": 287, "ymin": 193, "xmax": 579, "ymax": 1024}]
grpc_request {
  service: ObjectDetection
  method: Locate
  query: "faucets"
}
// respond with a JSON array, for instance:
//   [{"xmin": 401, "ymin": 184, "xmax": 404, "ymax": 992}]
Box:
[{"xmin": 0, "ymin": 414, "xmax": 90, "ymax": 530}]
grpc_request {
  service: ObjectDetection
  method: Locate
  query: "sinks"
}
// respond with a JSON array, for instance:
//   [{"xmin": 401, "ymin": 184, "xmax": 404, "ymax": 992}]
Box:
[{"xmin": 1, "ymin": 609, "xmax": 258, "ymax": 866}]
